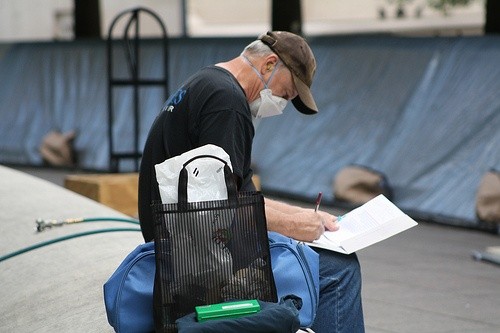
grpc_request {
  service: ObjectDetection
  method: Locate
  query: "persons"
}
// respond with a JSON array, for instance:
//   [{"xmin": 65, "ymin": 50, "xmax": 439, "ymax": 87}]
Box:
[{"xmin": 137, "ymin": 31, "xmax": 365, "ymax": 333}]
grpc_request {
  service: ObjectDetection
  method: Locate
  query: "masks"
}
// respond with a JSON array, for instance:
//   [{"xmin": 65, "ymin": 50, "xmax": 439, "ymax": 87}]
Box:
[{"xmin": 248, "ymin": 64, "xmax": 289, "ymax": 119}]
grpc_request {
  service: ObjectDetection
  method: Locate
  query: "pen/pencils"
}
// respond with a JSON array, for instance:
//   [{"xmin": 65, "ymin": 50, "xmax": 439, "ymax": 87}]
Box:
[{"xmin": 315, "ymin": 192, "xmax": 322, "ymax": 213}]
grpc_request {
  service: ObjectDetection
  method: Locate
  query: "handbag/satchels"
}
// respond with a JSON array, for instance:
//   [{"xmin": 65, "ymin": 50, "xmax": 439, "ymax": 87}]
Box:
[
  {"xmin": 103, "ymin": 230, "xmax": 320, "ymax": 333},
  {"xmin": 150, "ymin": 154, "xmax": 278, "ymax": 333}
]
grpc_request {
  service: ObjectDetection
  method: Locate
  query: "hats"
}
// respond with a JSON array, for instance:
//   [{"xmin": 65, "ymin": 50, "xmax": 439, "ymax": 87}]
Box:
[{"xmin": 258, "ymin": 31, "xmax": 319, "ymax": 115}]
